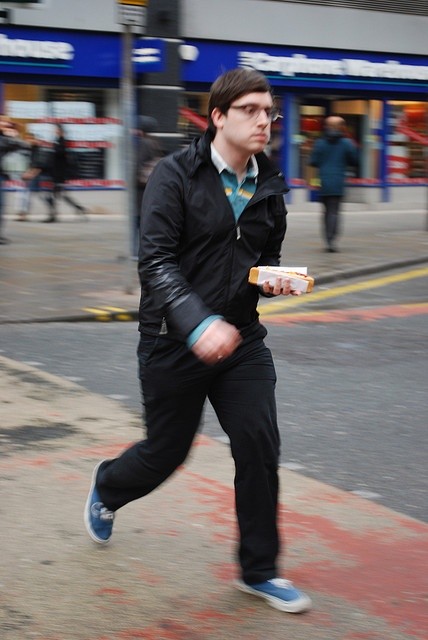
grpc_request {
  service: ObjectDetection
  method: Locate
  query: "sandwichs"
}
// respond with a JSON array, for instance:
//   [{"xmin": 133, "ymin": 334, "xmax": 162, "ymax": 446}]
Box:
[{"xmin": 249, "ymin": 266, "xmax": 314, "ymax": 292}]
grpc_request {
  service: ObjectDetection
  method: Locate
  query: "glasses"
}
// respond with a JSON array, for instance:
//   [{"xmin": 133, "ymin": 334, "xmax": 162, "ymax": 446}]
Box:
[{"xmin": 230, "ymin": 103, "xmax": 280, "ymax": 122}]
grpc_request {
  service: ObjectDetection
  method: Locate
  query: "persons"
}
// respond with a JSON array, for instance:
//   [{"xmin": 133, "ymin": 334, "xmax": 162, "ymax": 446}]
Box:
[
  {"xmin": 129, "ymin": 114, "xmax": 167, "ymax": 261},
  {"xmin": 305, "ymin": 115, "xmax": 358, "ymax": 253},
  {"xmin": 84, "ymin": 70, "xmax": 311, "ymax": 614},
  {"xmin": 0, "ymin": 115, "xmax": 84, "ymax": 246}
]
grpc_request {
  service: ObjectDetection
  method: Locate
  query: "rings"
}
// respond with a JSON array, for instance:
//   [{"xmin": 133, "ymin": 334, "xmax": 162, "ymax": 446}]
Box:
[{"xmin": 217, "ymin": 354, "xmax": 224, "ymax": 360}]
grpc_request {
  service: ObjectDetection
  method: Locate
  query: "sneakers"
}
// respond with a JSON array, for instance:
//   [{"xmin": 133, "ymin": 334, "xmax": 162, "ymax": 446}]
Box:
[
  {"xmin": 234, "ymin": 574, "xmax": 311, "ymax": 614},
  {"xmin": 84, "ymin": 458, "xmax": 115, "ymax": 545}
]
